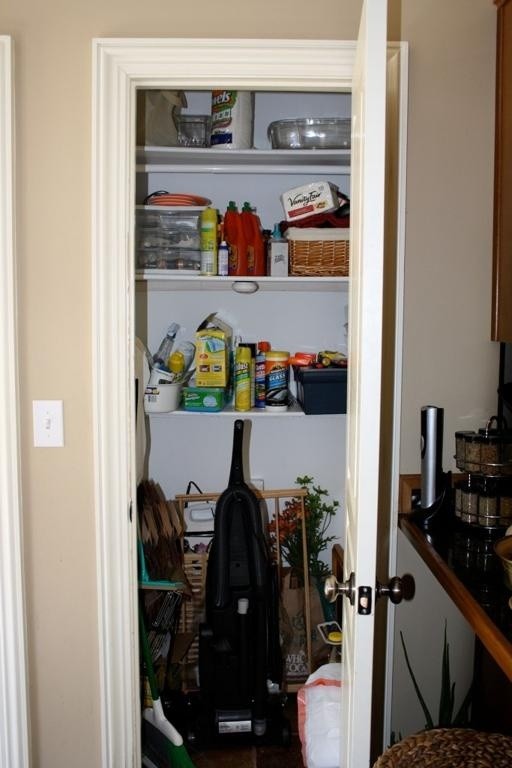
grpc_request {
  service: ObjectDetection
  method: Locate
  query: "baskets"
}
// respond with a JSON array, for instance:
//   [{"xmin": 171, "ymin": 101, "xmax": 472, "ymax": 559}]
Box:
[
  {"xmin": 284, "ymin": 225, "xmax": 352, "ymax": 277},
  {"xmin": 371, "ymin": 726, "xmax": 512, "ymax": 768}
]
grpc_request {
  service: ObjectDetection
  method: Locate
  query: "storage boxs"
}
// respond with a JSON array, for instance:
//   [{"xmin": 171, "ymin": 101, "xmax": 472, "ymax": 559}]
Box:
[{"xmin": 292, "ymin": 362, "xmax": 347, "ymax": 415}]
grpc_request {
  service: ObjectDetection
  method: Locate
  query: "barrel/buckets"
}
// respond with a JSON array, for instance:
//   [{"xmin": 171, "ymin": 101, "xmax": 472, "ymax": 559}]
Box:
[
  {"xmin": 239, "ymin": 202, "xmax": 266, "ymax": 276},
  {"xmin": 223, "ymin": 201, "xmax": 248, "ymax": 277}
]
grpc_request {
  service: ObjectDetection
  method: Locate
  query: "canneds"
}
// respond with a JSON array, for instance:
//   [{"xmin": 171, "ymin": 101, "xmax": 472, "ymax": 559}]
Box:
[{"xmin": 455, "ymin": 428, "xmax": 512, "ymax": 526}]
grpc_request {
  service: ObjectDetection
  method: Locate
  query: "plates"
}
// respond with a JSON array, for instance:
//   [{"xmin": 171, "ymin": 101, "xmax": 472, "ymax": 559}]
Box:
[{"xmin": 266, "ymin": 116, "xmax": 352, "ymax": 150}]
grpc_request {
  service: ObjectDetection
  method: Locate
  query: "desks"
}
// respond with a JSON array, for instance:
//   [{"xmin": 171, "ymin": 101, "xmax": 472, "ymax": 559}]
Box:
[{"xmin": 399, "ymin": 511, "xmax": 512, "ymax": 682}]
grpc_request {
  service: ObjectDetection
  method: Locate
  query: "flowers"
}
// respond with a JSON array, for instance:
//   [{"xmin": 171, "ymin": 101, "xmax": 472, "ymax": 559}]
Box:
[{"xmin": 267, "ymin": 474, "xmax": 342, "ymax": 617}]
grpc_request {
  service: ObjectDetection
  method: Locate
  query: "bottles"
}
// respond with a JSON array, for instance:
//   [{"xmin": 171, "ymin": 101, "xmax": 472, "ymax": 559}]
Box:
[
  {"xmin": 210, "ymin": 90, "xmax": 254, "ymax": 150},
  {"xmin": 454, "ymin": 426, "xmax": 512, "ymax": 528},
  {"xmin": 175, "ymin": 115, "xmax": 211, "ymax": 148},
  {"xmin": 311, "ymin": 633, "xmax": 342, "ymax": 675},
  {"xmin": 197, "ymin": 208, "xmax": 227, "ymax": 278},
  {"xmin": 235, "ymin": 339, "xmax": 290, "ymax": 411},
  {"xmin": 154, "ymin": 324, "xmax": 197, "ymax": 377}
]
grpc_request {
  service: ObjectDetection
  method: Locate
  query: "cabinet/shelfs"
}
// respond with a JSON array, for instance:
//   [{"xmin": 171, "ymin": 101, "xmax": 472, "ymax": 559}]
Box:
[{"xmin": 137, "ymin": 144, "xmax": 351, "ymax": 419}]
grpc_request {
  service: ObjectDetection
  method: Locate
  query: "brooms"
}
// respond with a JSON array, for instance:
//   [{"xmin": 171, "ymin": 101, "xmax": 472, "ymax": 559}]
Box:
[{"xmin": 141, "ymin": 599, "xmax": 197, "ymax": 768}]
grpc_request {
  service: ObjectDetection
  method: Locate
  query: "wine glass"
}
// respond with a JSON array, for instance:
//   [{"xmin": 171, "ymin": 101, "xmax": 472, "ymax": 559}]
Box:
[{"xmin": 494, "ymin": 534, "xmax": 512, "ymax": 609}]
